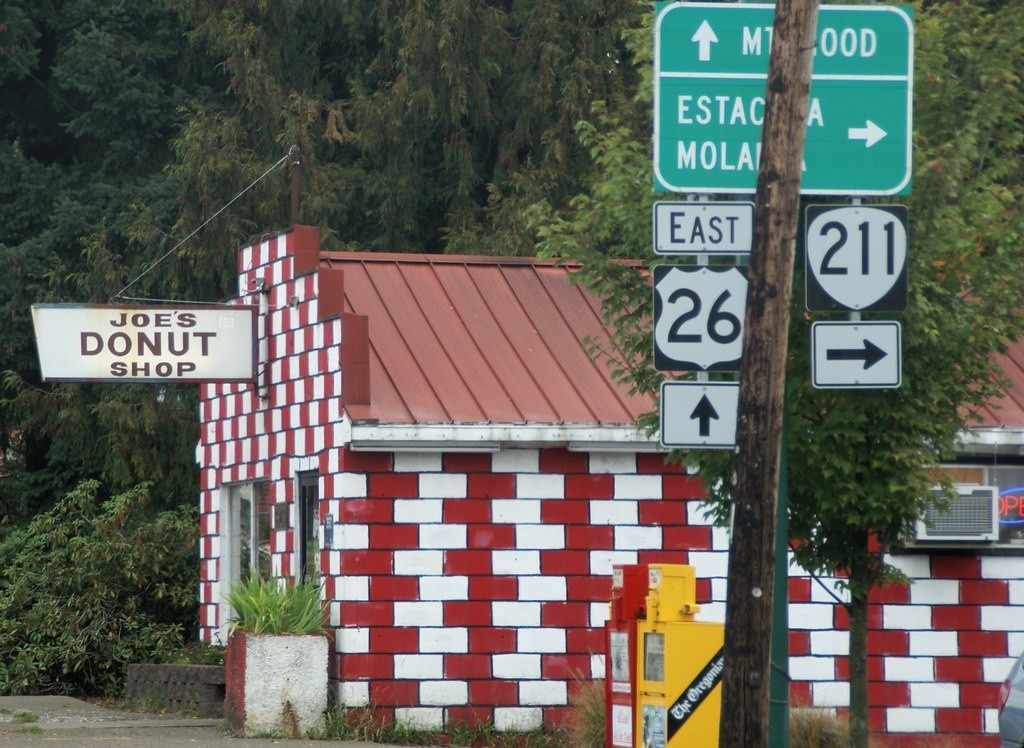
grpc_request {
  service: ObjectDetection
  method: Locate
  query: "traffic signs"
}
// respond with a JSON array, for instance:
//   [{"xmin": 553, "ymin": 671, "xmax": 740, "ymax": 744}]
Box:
[{"xmin": 653, "ymin": 2, "xmax": 916, "ymax": 195}]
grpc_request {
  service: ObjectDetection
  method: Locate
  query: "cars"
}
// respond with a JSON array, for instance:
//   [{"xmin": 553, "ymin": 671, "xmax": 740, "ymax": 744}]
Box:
[{"xmin": 997, "ymin": 649, "xmax": 1024, "ymax": 748}]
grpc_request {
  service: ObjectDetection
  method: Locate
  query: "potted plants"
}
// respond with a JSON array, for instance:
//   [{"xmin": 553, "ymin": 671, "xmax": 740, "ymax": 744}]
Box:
[{"xmin": 221, "ymin": 562, "xmax": 336, "ymax": 738}]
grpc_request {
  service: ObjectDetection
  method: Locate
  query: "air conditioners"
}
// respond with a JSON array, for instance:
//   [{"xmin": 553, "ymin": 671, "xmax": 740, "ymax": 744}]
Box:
[{"xmin": 907, "ymin": 484, "xmax": 999, "ymax": 541}]
